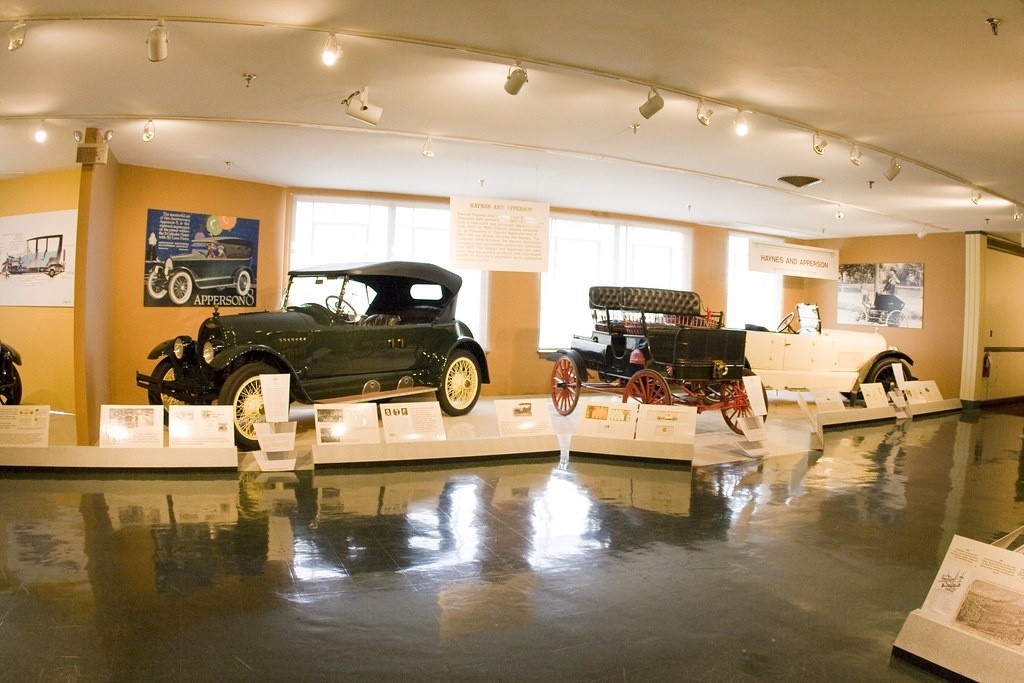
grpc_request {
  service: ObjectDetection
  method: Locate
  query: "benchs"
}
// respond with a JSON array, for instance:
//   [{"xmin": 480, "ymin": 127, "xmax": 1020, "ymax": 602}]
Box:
[
  {"xmin": 618, "ymin": 287, "xmax": 724, "ymax": 349},
  {"xmin": 589, "ymin": 287, "xmax": 641, "ymax": 334},
  {"xmin": 745, "ymin": 324, "xmax": 770, "ymax": 332},
  {"xmin": 389, "ymin": 305, "xmax": 443, "ymax": 325}
]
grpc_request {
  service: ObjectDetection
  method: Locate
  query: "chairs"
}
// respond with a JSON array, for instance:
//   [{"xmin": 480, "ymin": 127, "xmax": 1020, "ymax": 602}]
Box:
[{"xmin": 361, "ymin": 314, "xmax": 401, "ymax": 326}]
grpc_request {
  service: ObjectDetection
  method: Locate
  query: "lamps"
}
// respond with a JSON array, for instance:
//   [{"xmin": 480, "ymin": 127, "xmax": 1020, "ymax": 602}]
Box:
[
  {"xmin": 971, "ymin": 189, "xmax": 983, "ymax": 204},
  {"xmin": 917, "ymin": 224, "xmax": 927, "ymax": 238},
  {"xmin": 813, "ymin": 134, "xmax": 828, "ymax": 155},
  {"xmin": 146, "ymin": 19, "xmax": 170, "ymax": 62},
  {"xmin": 423, "ymin": 137, "xmax": 434, "ymax": 157},
  {"xmin": 8, "ymin": 20, "xmax": 27, "ymax": 51},
  {"xmin": 883, "ymin": 158, "xmax": 901, "ymax": 181},
  {"xmin": 697, "ymin": 98, "xmax": 714, "ymax": 126},
  {"xmin": 323, "ymin": 33, "xmax": 343, "ymax": 66},
  {"xmin": 505, "ymin": 59, "xmax": 528, "ymax": 95},
  {"xmin": 639, "ymin": 85, "xmax": 664, "ymax": 119},
  {"xmin": 850, "ymin": 143, "xmax": 862, "ymax": 166},
  {"xmin": 341, "ymin": 86, "xmax": 383, "ymax": 126},
  {"xmin": 142, "ymin": 119, "xmax": 154, "ymax": 142},
  {"xmin": 74, "ymin": 130, "xmax": 113, "ymax": 165}
]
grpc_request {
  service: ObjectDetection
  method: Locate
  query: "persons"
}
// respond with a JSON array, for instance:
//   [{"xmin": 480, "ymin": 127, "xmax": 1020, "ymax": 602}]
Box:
[{"xmin": 883, "ymin": 267, "xmax": 900, "ymax": 293}]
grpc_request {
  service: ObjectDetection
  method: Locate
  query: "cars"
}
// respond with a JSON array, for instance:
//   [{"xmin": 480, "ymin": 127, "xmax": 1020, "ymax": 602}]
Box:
[
  {"xmin": 726, "ymin": 301, "xmax": 919, "ymax": 406},
  {"xmin": 2, "ymin": 234, "xmax": 67, "ymax": 277},
  {"xmin": 135, "ymin": 261, "xmax": 490, "ymax": 452},
  {"xmin": 0, "ymin": 342, "xmax": 22, "ymax": 405},
  {"xmin": 142, "ymin": 237, "xmax": 254, "ymax": 305}
]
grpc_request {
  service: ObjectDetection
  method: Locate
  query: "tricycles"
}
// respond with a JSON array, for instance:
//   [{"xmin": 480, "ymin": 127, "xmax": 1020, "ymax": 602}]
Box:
[{"xmin": 848, "ymin": 285, "xmax": 908, "ymax": 327}]
